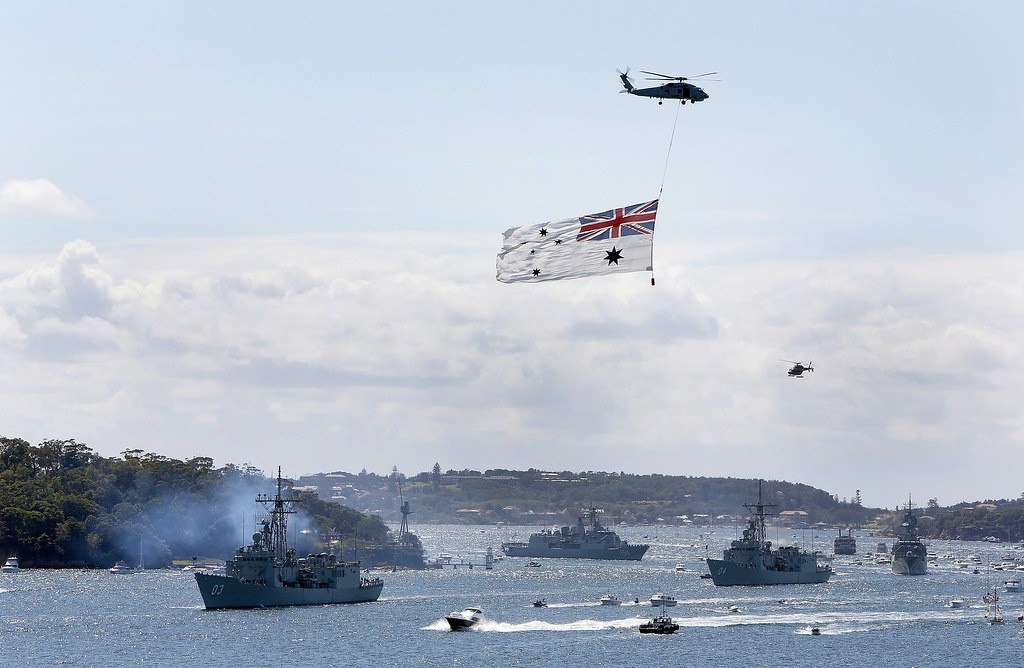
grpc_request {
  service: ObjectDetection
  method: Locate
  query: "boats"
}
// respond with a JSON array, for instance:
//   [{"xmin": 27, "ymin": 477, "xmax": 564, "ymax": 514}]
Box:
[
  {"xmin": 983, "ymin": 591, "xmax": 999, "ymax": 603},
  {"xmin": 834, "ymin": 529, "xmax": 856, "ymax": 555},
  {"xmin": 183, "ymin": 566, "xmax": 191, "ymax": 572},
  {"xmin": 194, "ymin": 466, "xmax": 384, "ymax": 609},
  {"xmin": 951, "ymin": 599, "xmax": 964, "ymax": 608},
  {"xmin": 1003, "ymin": 577, "xmax": 1024, "ymax": 592},
  {"xmin": 700, "ymin": 574, "xmax": 712, "ymax": 579},
  {"xmin": 601, "ymin": 595, "xmax": 622, "ymax": 605},
  {"xmin": 135, "ymin": 534, "xmax": 144, "ymax": 573},
  {"xmin": 811, "ymin": 628, "xmax": 821, "ymax": 635},
  {"xmin": 533, "ymin": 598, "xmax": 548, "ymax": 608},
  {"xmin": 639, "ymin": 602, "xmax": 679, "ymax": 634},
  {"xmin": 852, "ymin": 535, "xmax": 1024, "ymax": 573},
  {"xmin": 649, "ymin": 593, "xmax": 678, "ymax": 606},
  {"xmin": 525, "ymin": 562, "xmax": 541, "ymax": 567},
  {"xmin": 436, "ymin": 553, "xmax": 451, "ymax": 562},
  {"xmin": 445, "ymin": 607, "xmax": 483, "ymax": 631},
  {"xmin": 109, "ymin": 560, "xmax": 134, "ymax": 574},
  {"xmin": 707, "ymin": 480, "xmax": 835, "ymax": 586},
  {"xmin": 501, "ymin": 509, "xmax": 650, "ymax": 561},
  {"xmin": 1, "ymin": 557, "xmax": 22, "ymax": 572},
  {"xmin": 990, "ymin": 587, "xmax": 1005, "ymax": 626},
  {"xmin": 890, "ymin": 493, "xmax": 927, "ymax": 575},
  {"xmin": 728, "ymin": 605, "xmax": 738, "ymax": 611},
  {"xmin": 676, "ymin": 564, "xmax": 687, "ymax": 571}
]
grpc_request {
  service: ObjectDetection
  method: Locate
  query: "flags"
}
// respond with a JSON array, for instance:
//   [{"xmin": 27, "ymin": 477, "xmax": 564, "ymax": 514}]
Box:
[{"xmin": 497, "ymin": 200, "xmax": 660, "ymax": 284}]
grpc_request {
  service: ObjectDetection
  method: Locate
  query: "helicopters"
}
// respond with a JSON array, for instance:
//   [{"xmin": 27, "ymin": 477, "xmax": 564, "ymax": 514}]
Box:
[
  {"xmin": 617, "ymin": 67, "xmax": 721, "ymax": 105},
  {"xmin": 780, "ymin": 358, "xmax": 814, "ymax": 378}
]
